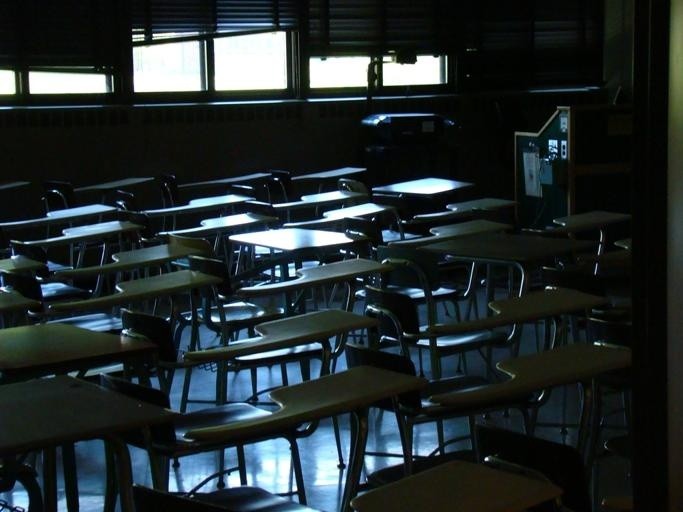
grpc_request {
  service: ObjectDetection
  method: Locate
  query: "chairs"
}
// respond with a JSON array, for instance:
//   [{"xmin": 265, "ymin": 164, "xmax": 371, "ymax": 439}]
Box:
[{"xmin": 0, "ymin": 164, "xmax": 632, "ymax": 511}]
